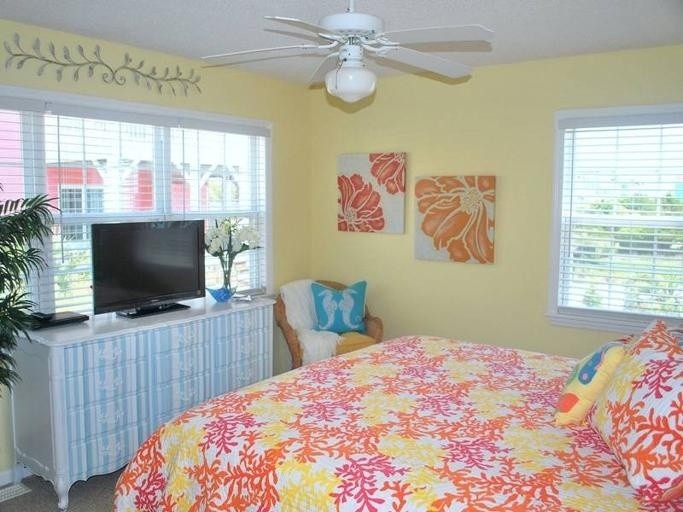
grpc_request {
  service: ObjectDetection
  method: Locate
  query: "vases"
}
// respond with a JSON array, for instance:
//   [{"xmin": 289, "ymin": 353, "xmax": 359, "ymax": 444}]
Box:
[{"xmin": 219, "ymin": 259, "xmax": 234, "ymax": 288}]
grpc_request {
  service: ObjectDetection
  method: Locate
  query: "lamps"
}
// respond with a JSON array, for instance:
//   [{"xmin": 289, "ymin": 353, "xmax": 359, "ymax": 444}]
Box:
[{"xmin": 322, "ymin": 59, "xmax": 376, "ymax": 104}]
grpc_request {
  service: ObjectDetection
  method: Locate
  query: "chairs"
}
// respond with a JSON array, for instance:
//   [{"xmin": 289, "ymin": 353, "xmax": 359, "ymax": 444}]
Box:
[{"xmin": 274, "ymin": 279, "xmax": 385, "ymax": 369}]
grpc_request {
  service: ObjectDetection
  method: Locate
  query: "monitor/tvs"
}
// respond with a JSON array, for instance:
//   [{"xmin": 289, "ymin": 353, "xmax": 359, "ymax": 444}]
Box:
[{"xmin": 92, "ymin": 220, "xmax": 205, "ymax": 319}]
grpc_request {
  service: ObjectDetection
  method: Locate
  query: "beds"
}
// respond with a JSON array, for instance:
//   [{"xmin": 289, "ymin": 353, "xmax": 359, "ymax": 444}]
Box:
[{"xmin": 113, "ymin": 328, "xmax": 683, "ymax": 511}]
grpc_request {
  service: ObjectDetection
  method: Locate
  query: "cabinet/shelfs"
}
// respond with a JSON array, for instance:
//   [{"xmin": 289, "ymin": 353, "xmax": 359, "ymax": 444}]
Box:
[{"xmin": 6, "ymin": 292, "xmax": 280, "ymax": 512}]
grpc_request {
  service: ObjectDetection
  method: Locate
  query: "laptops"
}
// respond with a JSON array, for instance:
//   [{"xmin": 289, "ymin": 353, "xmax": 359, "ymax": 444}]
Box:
[{"xmin": 18, "ymin": 312, "xmax": 89, "ymax": 331}]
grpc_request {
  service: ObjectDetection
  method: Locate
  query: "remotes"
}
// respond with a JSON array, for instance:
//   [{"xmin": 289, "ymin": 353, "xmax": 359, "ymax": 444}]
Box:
[{"xmin": 31, "ymin": 312, "xmax": 52, "ymax": 321}]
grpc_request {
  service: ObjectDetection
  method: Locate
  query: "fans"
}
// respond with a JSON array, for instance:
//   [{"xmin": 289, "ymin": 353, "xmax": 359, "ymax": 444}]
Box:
[{"xmin": 199, "ymin": 2, "xmax": 495, "ymax": 83}]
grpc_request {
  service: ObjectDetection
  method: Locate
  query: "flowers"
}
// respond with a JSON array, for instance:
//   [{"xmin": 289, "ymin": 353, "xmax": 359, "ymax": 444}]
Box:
[{"xmin": 203, "ymin": 217, "xmax": 264, "ymax": 288}]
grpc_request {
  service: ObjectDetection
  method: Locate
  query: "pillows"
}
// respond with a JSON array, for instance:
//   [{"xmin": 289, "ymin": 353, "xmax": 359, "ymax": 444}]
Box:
[
  {"xmin": 553, "ymin": 333, "xmax": 633, "ymax": 427},
  {"xmin": 309, "ymin": 281, "xmax": 370, "ymax": 333},
  {"xmin": 585, "ymin": 318, "xmax": 682, "ymax": 502}
]
